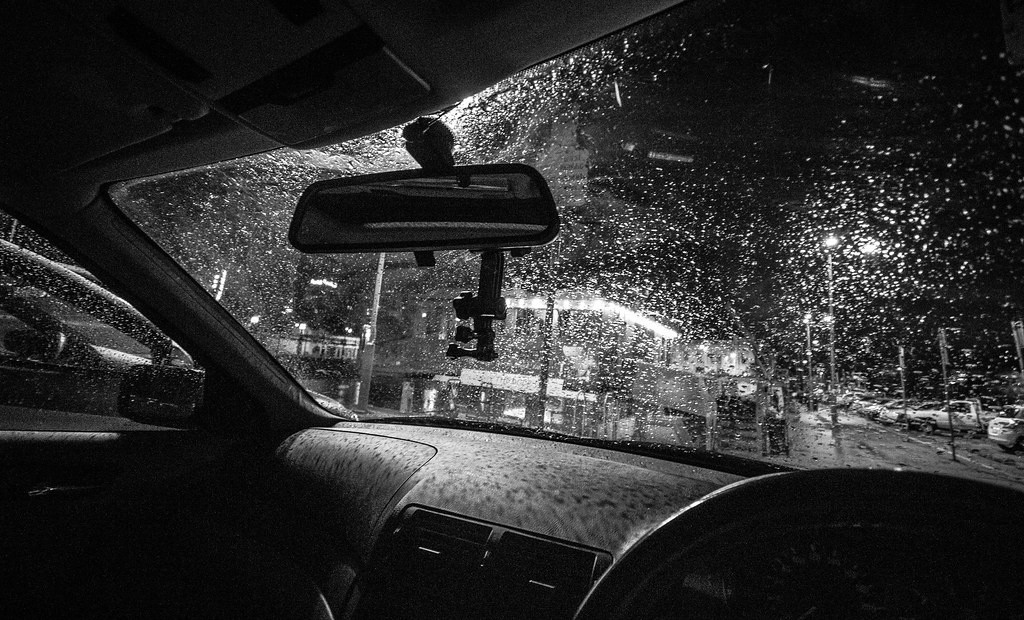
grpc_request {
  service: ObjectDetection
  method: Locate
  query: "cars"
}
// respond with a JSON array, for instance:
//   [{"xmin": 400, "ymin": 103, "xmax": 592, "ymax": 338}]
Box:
[
  {"xmin": 0, "ymin": 238, "xmax": 358, "ymax": 434},
  {"xmin": 987, "ymin": 404, "xmax": 1024, "ymax": 454},
  {"xmin": 813, "ymin": 381, "xmax": 1004, "ymax": 436}
]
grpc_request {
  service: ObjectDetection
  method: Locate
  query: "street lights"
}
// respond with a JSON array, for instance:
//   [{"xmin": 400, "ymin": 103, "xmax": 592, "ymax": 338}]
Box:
[
  {"xmin": 822, "ymin": 234, "xmax": 840, "ymax": 428},
  {"xmin": 802, "ymin": 314, "xmax": 815, "ymax": 414}
]
